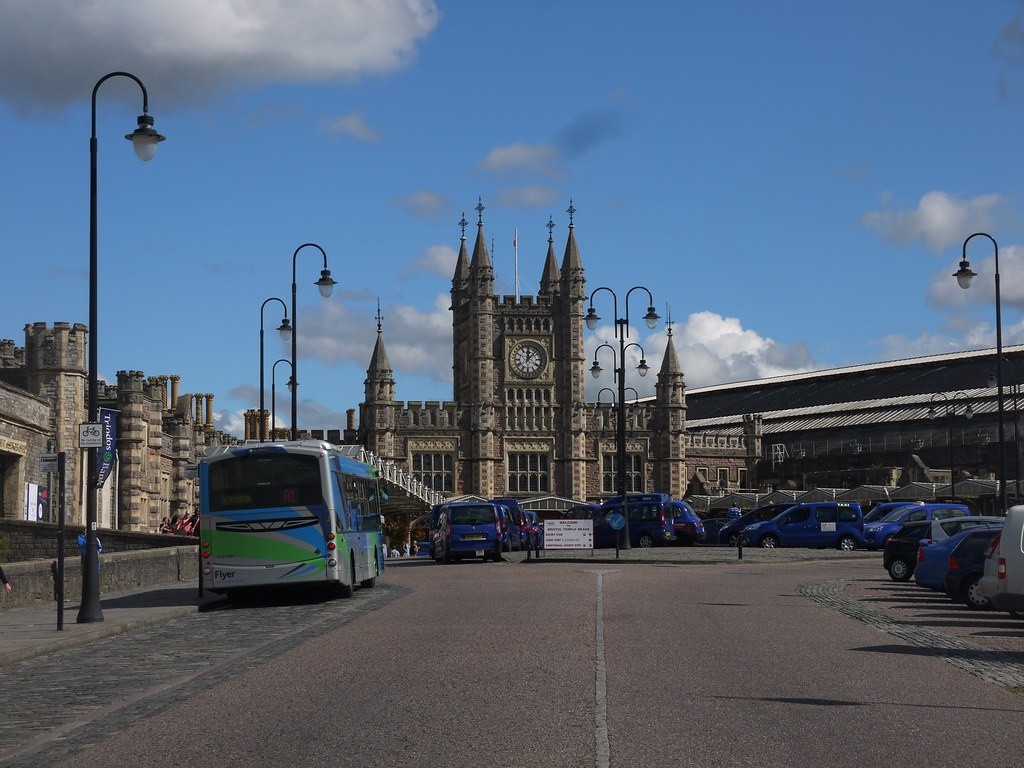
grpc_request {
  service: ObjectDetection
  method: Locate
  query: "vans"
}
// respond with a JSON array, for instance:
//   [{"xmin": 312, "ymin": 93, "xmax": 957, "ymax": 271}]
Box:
[
  {"xmin": 718, "ymin": 501, "xmax": 804, "ymax": 546},
  {"xmin": 740, "ymin": 499, "xmax": 865, "ymax": 551},
  {"xmin": 864, "ymin": 504, "xmax": 976, "ymax": 551},
  {"xmin": 417, "ymin": 492, "xmax": 730, "ymax": 563},
  {"xmin": 866, "ymin": 502, "xmax": 916, "ymax": 522}
]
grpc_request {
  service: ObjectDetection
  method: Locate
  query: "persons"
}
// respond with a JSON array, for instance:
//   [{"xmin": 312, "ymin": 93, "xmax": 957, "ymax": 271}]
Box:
[
  {"xmin": 402, "ymin": 538, "xmax": 411, "ymax": 558},
  {"xmin": 159, "ymin": 507, "xmax": 200, "ymax": 536},
  {"xmin": 391, "ymin": 545, "xmax": 400, "ymax": 558},
  {"xmin": 0, "ymin": 566, "xmax": 11, "ymax": 593},
  {"xmin": 413, "ymin": 541, "xmax": 420, "ymax": 557},
  {"xmin": 383, "ymin": 544, "xmax": 387, "ymax": 559},
  {"xmin": 76, "ymin": 527, "xmax": 103, "ymax": 596}
]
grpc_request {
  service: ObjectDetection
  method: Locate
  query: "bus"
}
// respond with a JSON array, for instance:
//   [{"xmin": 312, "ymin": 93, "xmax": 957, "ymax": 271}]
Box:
[{"xmin": 197, "ymin": 435, "xmax": 389, "ymax": 600}]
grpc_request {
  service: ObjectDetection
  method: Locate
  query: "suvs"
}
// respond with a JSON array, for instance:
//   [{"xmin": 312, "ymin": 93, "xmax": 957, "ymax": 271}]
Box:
[{"xmin": 882, "ymin": 504, "xmax": 1024, "ymax": 619}]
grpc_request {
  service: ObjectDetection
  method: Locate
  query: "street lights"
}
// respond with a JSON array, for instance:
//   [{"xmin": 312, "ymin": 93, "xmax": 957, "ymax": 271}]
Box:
[
  {"xmin": 926, "ymin": 390, "xmax": 974, "ymax": 503},
  {"xmin": 288, "ymin": 242, "xmax": 339, "ymax": 442},
  {"xmin": 257, "ymin": 297, "xmax": 293, "ymax": 443},
  {"xmin": 77, "ymin": 70, "xmax": 166, "ymax": 622},
  {"xmin": 950, "ymin": 231, "xmax": 1008, "ymax": 518},
  {"xmin": 581, "ymin": 285, "xmax": 661, "ymax": 550},
  {"xmin": 270, "ymin": 358, "xmax": 301, "ymax": 444}
]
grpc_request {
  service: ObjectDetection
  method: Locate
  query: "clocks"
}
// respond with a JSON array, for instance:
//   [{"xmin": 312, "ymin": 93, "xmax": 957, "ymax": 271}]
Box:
[{"xmin": 512, "ymin": 344, "xmax": 545, "ymax": 376}]
